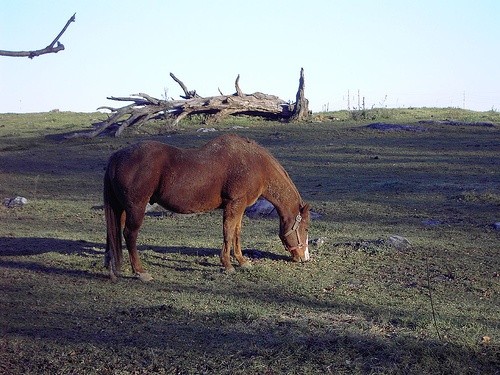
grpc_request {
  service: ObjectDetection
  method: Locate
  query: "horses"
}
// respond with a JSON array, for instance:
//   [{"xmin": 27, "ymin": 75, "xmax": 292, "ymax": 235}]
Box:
[{"xmin": 103, "ymin": 133, "xmax": 310, "ymax": 281}]
